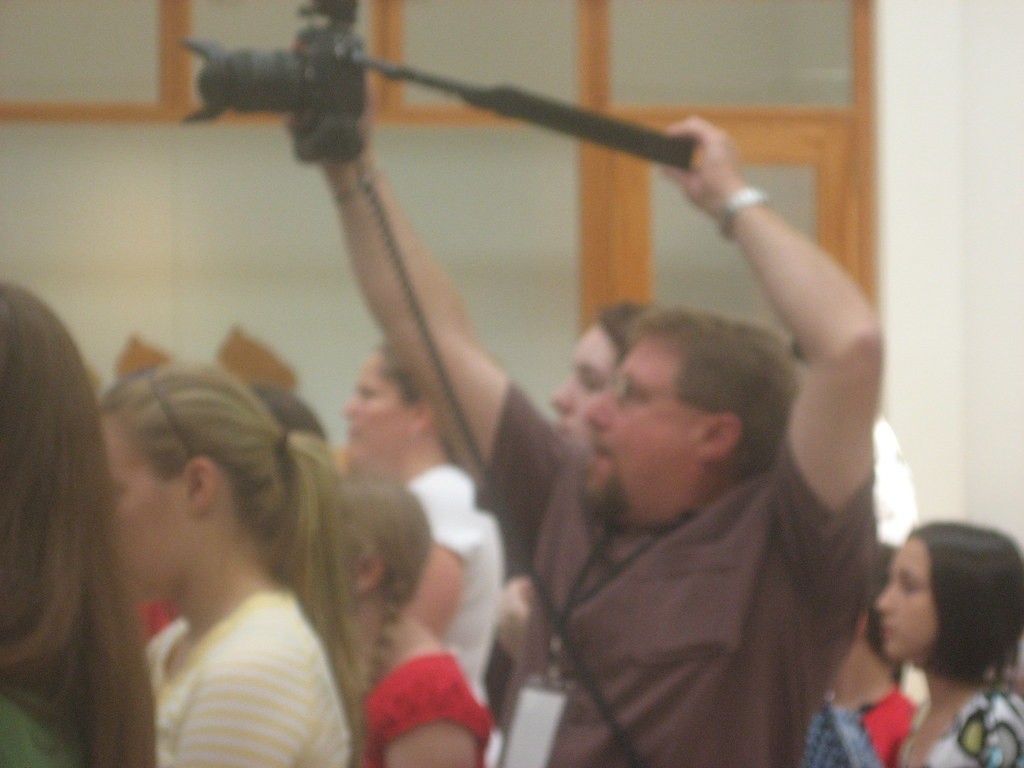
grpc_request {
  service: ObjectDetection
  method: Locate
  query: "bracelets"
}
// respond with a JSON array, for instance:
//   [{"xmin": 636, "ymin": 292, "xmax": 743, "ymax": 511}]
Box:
[{"xmin": 722, "ymin": 189, "xmax": 773, "ymax": 241}]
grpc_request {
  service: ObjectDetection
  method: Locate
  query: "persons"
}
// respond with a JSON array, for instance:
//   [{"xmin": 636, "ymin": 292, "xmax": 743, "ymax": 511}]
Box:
[
  {"xmin": 263, "ymin": 300, "xmax": 1023, "ymax": 767},
  {"xmin": 324, "ymin": 116, "xmax": 883, "ymax": 768},
  {"xmin": 103, "ymin": 369, "xmax": 365, "ymax": 768},
  {"xmin": 0, "ymin": 277, "xmax": 157, "ymax": 768}
]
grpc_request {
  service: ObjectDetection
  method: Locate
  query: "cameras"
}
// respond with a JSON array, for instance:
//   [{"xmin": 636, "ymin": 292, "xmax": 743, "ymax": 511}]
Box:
[{"xmin": 181, "ymin": 0, "xmax": 369, "ymax": 162}]
